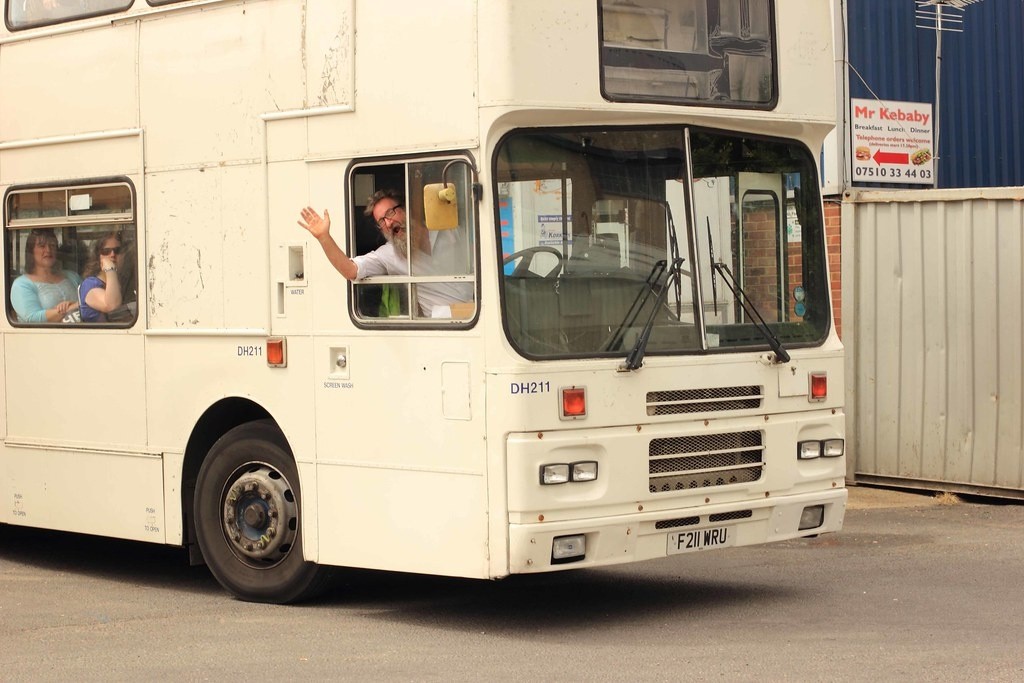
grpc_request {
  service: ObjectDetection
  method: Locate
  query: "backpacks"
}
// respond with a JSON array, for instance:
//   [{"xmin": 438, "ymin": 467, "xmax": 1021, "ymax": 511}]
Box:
[{"xmin": 105, "ymin": 284, "xmax": 136, "ymax": 322}]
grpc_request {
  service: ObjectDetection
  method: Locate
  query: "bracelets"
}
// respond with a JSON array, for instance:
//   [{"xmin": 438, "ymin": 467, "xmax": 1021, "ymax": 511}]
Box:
[{"xmin": 105, "ymin": 266, "xmax": 118, "ymax": 272}]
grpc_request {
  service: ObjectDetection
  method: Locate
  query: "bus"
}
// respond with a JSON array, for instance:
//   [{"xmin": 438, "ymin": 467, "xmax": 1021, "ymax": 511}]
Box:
[{"xmin": 0, "ymin": 1, "xmax": 849, "ymax": 604}]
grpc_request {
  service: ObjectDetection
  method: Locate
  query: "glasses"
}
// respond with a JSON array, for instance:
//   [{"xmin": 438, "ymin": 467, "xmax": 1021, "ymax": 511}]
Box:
[
  {"xmin": 98, "ymin": 246, "xmax": 123, "ymax": 256},
  {"xmin": 31, "ymin": 228, "xmax": 54, "ymax": 236},
  {"xmin": 374, "ymin": 203, "xmax": 402, "ymax": 229}
]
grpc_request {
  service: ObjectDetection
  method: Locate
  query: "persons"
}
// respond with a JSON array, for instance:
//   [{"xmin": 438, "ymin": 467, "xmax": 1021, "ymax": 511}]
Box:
[
  {"xmin": 10, "ymin": 229, "xmax": 83, "ymax": 324},
  {"xmin": 79, "ymin": 232, "xmax": 135, "ymax": 321},
  {"xmin": 298, "ymin": 190, "xmax": 474, "ymax": 318}
]
